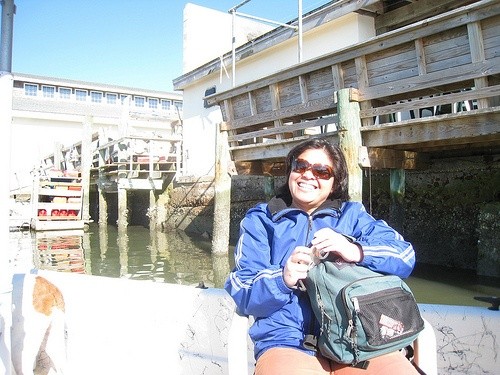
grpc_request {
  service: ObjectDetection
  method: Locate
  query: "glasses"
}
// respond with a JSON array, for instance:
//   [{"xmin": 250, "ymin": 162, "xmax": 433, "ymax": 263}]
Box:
[{"xmin": 290, "ymin": 158, "xmax": 334, "ymax": 180}]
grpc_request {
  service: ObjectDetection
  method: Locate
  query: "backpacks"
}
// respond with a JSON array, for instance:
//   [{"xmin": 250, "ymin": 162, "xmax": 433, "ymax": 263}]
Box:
[{"xmin": 302, "ymin": 250, "xmax": 424, "ymax": 364}]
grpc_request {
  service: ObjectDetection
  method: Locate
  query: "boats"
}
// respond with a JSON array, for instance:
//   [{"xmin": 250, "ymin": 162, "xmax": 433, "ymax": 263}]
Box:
[{"xmin": 1, "ymin": 265, "xmax": 499, "ymax": 375}]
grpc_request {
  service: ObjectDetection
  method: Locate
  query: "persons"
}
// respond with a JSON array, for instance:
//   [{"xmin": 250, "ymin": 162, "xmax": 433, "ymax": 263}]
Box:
[{"xmin": 223, "ymin": 138, "xmax": 431, "ymax": 375}]
